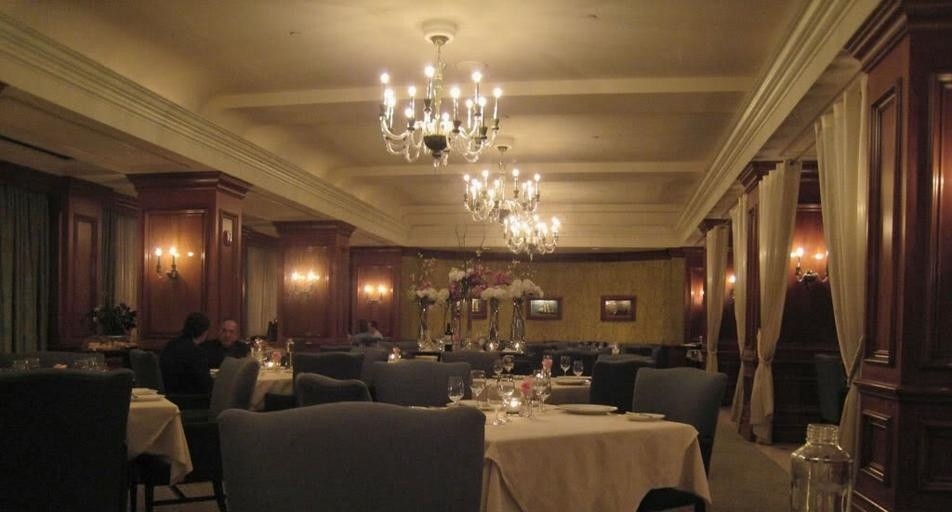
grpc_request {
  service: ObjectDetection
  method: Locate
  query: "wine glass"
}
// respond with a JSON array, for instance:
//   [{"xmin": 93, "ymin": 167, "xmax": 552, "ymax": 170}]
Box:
[
  {"xmin": 24, "ymin": 358, "xmax": 39, "ymax": 369},
  {"xmin": 446, "ymin": 354, "xmax": 553, "ymax": 425},
  {"xmin": 12, "ymin": 360, "xmax": 29, "ymax": 370},
  {"xmin": 573, "ymin": 360, "xmax": 584, "ymax": 376},
  {"xmin": 560, "ymin": 355, "xmax": 570, "ymax": 375}
]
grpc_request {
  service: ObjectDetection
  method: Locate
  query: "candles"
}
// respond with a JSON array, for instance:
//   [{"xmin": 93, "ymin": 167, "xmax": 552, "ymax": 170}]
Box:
[
  {"xmin": 384, "ymin": 88, "xmax": 390, "ymax": 115},
  {"xmin": 388, "ymin": 92, "xmax": 395, "ymax": 123},
  {"xmin": 409, "ymin": 87, "xmax": 415, "ymax": 118},
  {"xmin": 483, "ymin": 171, "xmax": 487, "ymax": 192},
  {"xmin": 535, "ymin": 174, "xmax": 540, "ymax": 196},
  {"xmin": 513, "ymin": 168, "xmax": 519, "ymax": 190},
  {"xmin": 473, "ymin": 179, "xmax": 476, "ymax": 199},
  {"xmin": 157, "ymin": 248, "xmax": 161, "ymax": 264},
  {"xmin": 465, "ymin": 175, "xmax": 470, "ymax": 195},
  {"xmin": 442, "ymin": 112, "xmax": 449, "ymax": 134},
  {"xmin": 380, "ymin": 72, "xmax": 387, "ymax": 104},
  {"xmin": 172, "ymin": 247, "xmax": 176, "ymax": 265},
  {"xmin": 494, "ymin": 89, "xmax": 501, "ymax": 119},
  {"xmin": 426, "ymin": 65, "xmax": 434, "ymax": 98},
  {"xmin": 475, "ymin": 72, "xmax": 480, "ymax": 104},
  {"xmin": 797, "ymin": 248, "xmax": 801, "ymax": 267},
  {"xmin": 480, "ymin": 97, "xmax": 485, "ymax": 127},
  {"xmin": 453, "ymin": 87, "xmax": 458, "ymax": 120},
  {"xmin": 466, "ymin": 99, "xmax": 473, "ymax": 129}
]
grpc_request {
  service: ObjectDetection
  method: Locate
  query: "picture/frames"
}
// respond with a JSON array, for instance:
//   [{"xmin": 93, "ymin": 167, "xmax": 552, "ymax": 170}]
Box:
[
  {"xmin": 601, "ymin": 295, "xmax": 636, "ymax": 321},
  {"xmin": 453, "ymin": 295, "xmax": 486, "ymax": 319},
  {"xmin": 526, "ymin": 294, "xmax": 562, "ymax": 320}
]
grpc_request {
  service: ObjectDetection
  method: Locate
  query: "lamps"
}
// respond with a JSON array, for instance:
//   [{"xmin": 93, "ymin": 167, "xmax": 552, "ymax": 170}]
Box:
[
  {"xmin": 794, "ymin": 247, "xmax": 829, "ymax": 292},
  {"xmin": 155, "ymin": 247, "xmax": 178, "ymax": 281},
  {"xmin": 503, "ymin": 211, "xmax": 561, "ymax": 261},
  {"xmin": 463, "ymin": 136, "xmax": 541, "ymax": 226},
  {"xmin": 378, "ymin": 19, "xmax": 502, "ymax": 169}
]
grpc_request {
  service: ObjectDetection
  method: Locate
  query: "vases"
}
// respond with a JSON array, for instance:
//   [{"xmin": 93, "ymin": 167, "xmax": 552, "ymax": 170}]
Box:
[
  {"xmin": 272, "ymin": 359, "xmax": 281, "ymax": 373},
  {"xmin": 543, "ymin": 366, "xmax": 551, "ymax": 378},
  {"xmin": 485, "ymin": 305, "xmax": 501, "ymax": 352},
  {"xmin": 510, "ymin": 303, "xmax": 525, "ymax": 353},
  {"xmin": 416, "ymin": 309, "xmax": 432, "ymax": 351},
  {"xmin": 448, "ymin": 301, "xmax": 462, "ymax": 352},
  {"xmin": 518, "ymin": 390, "xmax": 533, "ymax": 417},
  {"xmin": 460, "ymin": 298, "xmax": 473, "ymax": 351},
  {"xmin": 100, "ymin": 335, "xmax": 130, "ymax": 342}
]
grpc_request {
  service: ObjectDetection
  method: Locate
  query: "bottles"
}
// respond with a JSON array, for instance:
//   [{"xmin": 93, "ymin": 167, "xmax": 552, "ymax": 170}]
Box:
[
  {"xmin": 285, "ymin": 339, "xmax": 295, "ymax": 368},
  {"xmin": 445, "ymin": 323, "xmax": 452, "ymax": 351},
  {"xmin": 790, "ymin": 424, "xmax": 852, "ymax": 512}
]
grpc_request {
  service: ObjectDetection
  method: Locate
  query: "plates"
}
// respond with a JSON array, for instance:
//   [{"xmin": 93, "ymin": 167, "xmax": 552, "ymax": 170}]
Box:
[
  {"xmin": 554, "ymin": 380, "xmax": 587, "ymax": 385},
  {"xmin": 559, "ymin": 403, "xmax": 618, "ymax": 413}
]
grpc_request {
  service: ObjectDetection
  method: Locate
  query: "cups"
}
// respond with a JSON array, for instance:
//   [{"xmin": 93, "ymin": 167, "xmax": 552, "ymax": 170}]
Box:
[{"xmin": 272, "ymin": 351, "xmax": 281, "ymax": 369}]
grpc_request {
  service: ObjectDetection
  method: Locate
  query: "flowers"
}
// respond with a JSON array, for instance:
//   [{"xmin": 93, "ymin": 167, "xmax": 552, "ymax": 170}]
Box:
[
  {"xmin": 406, "ymin": 249, "xmax": 437, "ymax": 338},
  {"xmin": 88, "ymin": 302, "xmax": 137, "ymax": 336},
  {"xmin": 437, "ymin": 268, "xmax": 468, "ymax": 334},
  {"xmin": 542, "ymin": 358, "xmax": 553, "ymax": 379},
  {"xmin": 448, "ymin": 225, "xmax": 492, "ymax": 326},
  {"xmin": 480, "ymin": 271, "xmax": 513, "ymax": 331},
  {"xmin": 272, "ymin": 352, "xmax": 281, "ymax": 363},
  {"xmin": 515, "ymin": 375, "xmax": 534, "ymax": 406},
  {"xmin": 507, "ymin": 258, "xmax": 544, "ymax": 331}
]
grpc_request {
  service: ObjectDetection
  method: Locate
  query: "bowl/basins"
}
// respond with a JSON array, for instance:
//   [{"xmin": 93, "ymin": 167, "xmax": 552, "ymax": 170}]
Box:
[{"xmin": 623, "ymin": 413, "xmax": 665, "ymax": 421}]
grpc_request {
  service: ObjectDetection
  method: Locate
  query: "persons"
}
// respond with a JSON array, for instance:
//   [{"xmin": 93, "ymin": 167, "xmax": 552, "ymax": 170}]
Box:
[
  {"xmin": 364, "ymin": 319, "xmax": 382, "ymax": 339},
  {"xmin": 205, "ymin": 317, "xmax": 252, "ymax": 369},
  {"xmin": 160, "ymin": 311, "xmax": 211, "ymax": 409}
]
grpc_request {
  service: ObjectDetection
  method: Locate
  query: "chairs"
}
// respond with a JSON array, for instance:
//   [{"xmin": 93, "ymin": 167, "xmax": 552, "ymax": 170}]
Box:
[
  {"xmin": 544, "ymin": 349, "xmax": 598, "ymax": 377},
  {"xmin": 372, "ymin": 360, "xmax": 473, "ymax": 410},
  {"xmin": 0, "ymin": 367, "xmax": 133, "ymax": 512},
  {"xmin": 442, "ymin": 351, "xmax": 501, "ymax": 378},
  {"xmin": 501, "ymin": 342, "xmax": 555, "ymax": 375},
  {"xmin": 129, "ymin": 348, "xmax": 164, "ymax": 393},
  {"xmin": 590, "ymin": 359, "xmax": 655, "ymax": 417},
  {"xmin": 283, "ymin": 335, "xmax": 417, "ymax": 359},
  {"xmin": 632, "ymin": 368, "xmax": 727, "ymax": 512},
  {"xmin": 651, "ymin": 344, "xmax": 687, "ymax": 367},
  {"xmin": 291, "ymin": 351, "xmax": 365, "ymax": 381},
  {"xmin": 294, "ymin": 373, "xmax": 372, "ymax": 408},
  {"xmin": 130, "ymin": 356, "xmax": 260, "ymax": 512},
  {"xmin": 217, "ymin": 401, "xmax": 485, "ymax": 512}
]
